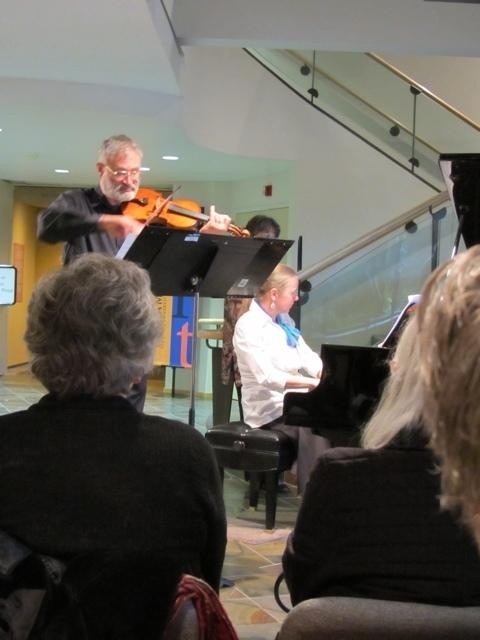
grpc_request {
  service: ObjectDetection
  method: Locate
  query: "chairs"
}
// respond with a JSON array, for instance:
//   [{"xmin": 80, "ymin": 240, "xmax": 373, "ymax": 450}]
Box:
[{"xmin": 275, "ymin": 596, "xmax": 480, "ymax": 640}]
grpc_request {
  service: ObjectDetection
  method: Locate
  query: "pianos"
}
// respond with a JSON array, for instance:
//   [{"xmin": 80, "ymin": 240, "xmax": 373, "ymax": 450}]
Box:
[{"xmin": 281, "ymin": 152, "xmax": 480, "ymax": 447}]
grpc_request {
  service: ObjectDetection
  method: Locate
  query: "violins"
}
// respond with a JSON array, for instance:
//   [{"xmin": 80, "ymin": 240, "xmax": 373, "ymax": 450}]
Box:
[{"xmin": 121, "ymin": 186, "xmax": 251, "ymax": 238}]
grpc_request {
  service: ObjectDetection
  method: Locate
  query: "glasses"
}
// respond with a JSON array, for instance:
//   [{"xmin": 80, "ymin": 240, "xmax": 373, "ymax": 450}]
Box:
[{"xmin": 105, "ymin": 164, "xmax": 141, "ymax": 180}]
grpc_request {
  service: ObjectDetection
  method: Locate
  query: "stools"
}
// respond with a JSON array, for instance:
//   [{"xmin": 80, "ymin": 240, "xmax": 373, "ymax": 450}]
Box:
[{"xmin": 204, "ymin": 419, "xmax": 297, "ymax": 529}]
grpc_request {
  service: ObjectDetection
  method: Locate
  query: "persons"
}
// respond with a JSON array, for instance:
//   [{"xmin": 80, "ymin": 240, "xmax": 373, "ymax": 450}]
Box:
[
  {"xmin": 35, "ymin": 134, "xmax": 231, "ymax": 418},
  {"xmin": 278, "ymin": 243, "xmax": 480, "ymax": 609},
  {"xmin": 1, "ymin": 252, "xmax": 230, "ymax": 639},
  {"xmin": 217, "ymin": 214, "xmax": 282, "ymax": 423},
  {"xmin": 230, "ymin": 261, "xmax": 338, "ymax": 504}
]
